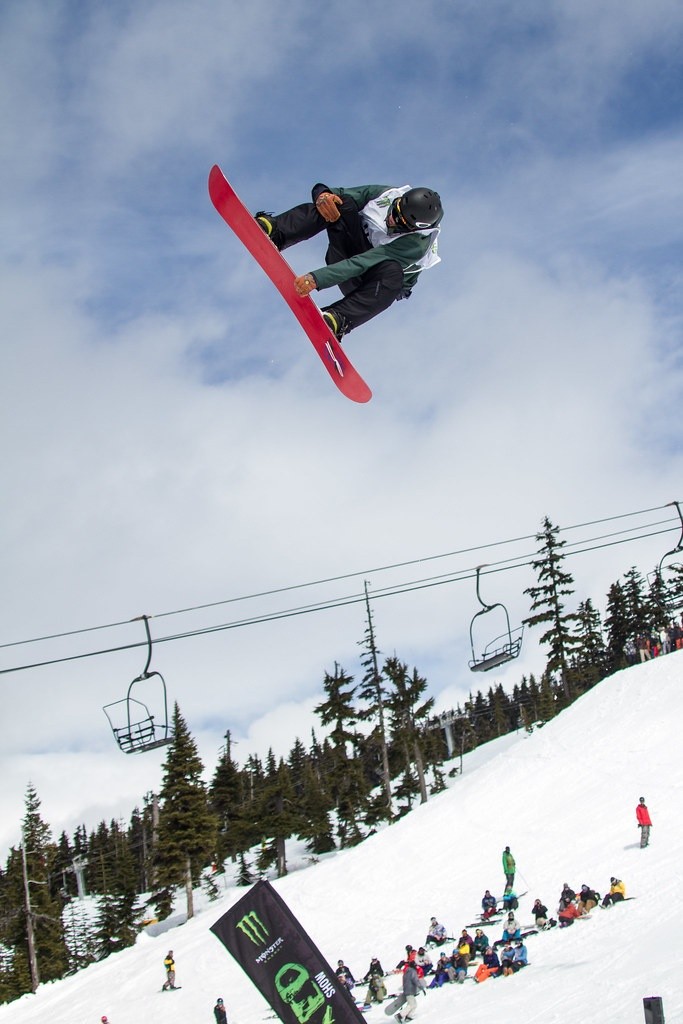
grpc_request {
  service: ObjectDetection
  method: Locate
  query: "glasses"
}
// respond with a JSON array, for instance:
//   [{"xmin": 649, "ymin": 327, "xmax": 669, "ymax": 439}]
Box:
[{"xmin": 392, "ymin": 202, "xmax": 412, "ymax": 232}]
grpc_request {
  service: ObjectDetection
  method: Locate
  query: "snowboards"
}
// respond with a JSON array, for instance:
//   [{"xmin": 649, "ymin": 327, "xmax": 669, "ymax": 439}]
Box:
[
  {"xmin": 159, "ymin": 986, "xmax": 183, "ymax": 993},
  {"xmin": 384, "ymin": 992, "xmax": 418, "ymax": 1015},
  {"xmin": 206, "ymin": 163, "xmax": 373, "ymax": 404}
]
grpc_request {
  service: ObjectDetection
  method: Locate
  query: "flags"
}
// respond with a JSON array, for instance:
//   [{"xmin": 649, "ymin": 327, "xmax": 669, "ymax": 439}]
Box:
[{"xmin": 211, "ymin": 878, "xmax": 368, "ymax": 1024}]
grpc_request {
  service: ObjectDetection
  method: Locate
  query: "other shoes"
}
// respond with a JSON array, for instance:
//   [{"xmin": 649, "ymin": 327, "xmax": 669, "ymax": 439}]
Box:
[
  {"xmin": 395, "ymin": 1014, "xmax": 402, "ymax": 1024},
  {"xmin": 323, "ymin": 308, "xmax": 342, "ymax": 336},
  {"xmin": 255, "ymin": 214, "xmax": 277, "ymax": 236},
  {"xmin": 405, "ymin": 1016, "xmax": 413, "ymax": 1022}
]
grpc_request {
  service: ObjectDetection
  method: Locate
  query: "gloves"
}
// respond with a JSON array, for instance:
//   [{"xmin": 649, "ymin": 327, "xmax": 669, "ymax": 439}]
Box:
[
  {"xmin": 315, "ymin": 192, "xmax": 343, "ymax": 222},
  {"xmin": 294, "ymin": 273, "xmax": 319, "ymax": 297}
]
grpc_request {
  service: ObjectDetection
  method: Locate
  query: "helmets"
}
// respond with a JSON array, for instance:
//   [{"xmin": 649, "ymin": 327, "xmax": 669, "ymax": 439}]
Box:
[{"xmin": 400, "ymin": 188, "xmax": 442, "ymax": 229}]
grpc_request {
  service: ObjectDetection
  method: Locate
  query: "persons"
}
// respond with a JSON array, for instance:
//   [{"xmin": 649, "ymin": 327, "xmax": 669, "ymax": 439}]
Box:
[
  {"xmin": 162, "ymin": 950, "xmax": 176, "ymax": 991},
  {"xmin": 531, "ymin": 899, "xmax": 557, "ymax": 930},
  {"xmin": 501, "ymin": 846, "xmax": 516, "ymax": 891},
  {"xmin": 474, "ymin": 938, "xmax": 527, "ymax": 983},
  {"xmin": 102, "ymin": 1016, "xmax": 110, "ymax": 1024},
  {"xmin": 637, "ymin": 613, "xmax": 683, "ymax": 662},
  {"xmin": 214, "ymin": 998, "xmax": 227, "ymax": 1024},
  {"xmin": 556, "ymin": 876, "xmax": 626, "ymax": 928},
  {"xmin": 426, "ymin": 948, "xmax": 467, "ymax": 990},
  {"xmin": 337, "ymin": 958, "xmax": 387, "ymax": 1009},
  {"xmin": 424, "ymin": 917, "xmax": 447, "ymax": 949},
  {"xmin": 636, "ymin": 796, "xmax": 652, "ymax": 848},
  {"xmin": 457, "ymin": 929, "xmax": 488, "ymax": 966},
  {"xmin": 481, "ymin": 890, "xmax": 497, "ymax": 922},
  {"xmin": 394, "ymin": 945, "xmax": 433, "ymax": 977},
  {"xmin": 502, "ymin": 911, "xmax": 521, "ymax": 945},
  {"xmin": 254, "ymin": 183, "xmax": 445, "ymax": 343},
  {"xmin": 502, "ymin": 884, "xmax": 519, "ymax": 911},
  {"xmin": 394, "ymin": 960, "xmax": 427, "ymax": 1024}
]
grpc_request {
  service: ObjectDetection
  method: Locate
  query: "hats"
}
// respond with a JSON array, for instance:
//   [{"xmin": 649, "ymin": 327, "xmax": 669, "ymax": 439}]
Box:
[
  {"xmin": 372, "ymin": 957, "xmax": 377, "ymax": 960},
  {"xmin": 453, "ymin": 950, "xmax": 458, "ymax": 954},
  {"xmin": 217, "ymin": 998, "xmax": 223, "ymax": 1002},
  {"xmin": 441, "ymin": 953, "xmax": 445, "ymax": 957},
  {"xmin": 338, "ymin": 960, "xmax": 343, "ymax": 965},
  {"xmin": 409, "ymin": 961, "xmax": 417, "ymax": 967},
  {"xmin": 102, "ymin": 1016, "xmax": 107, "ymax": 1020},
  {"xmin": 406, "ymin": 945, "xmax": 411, "ymax": 950}
]
